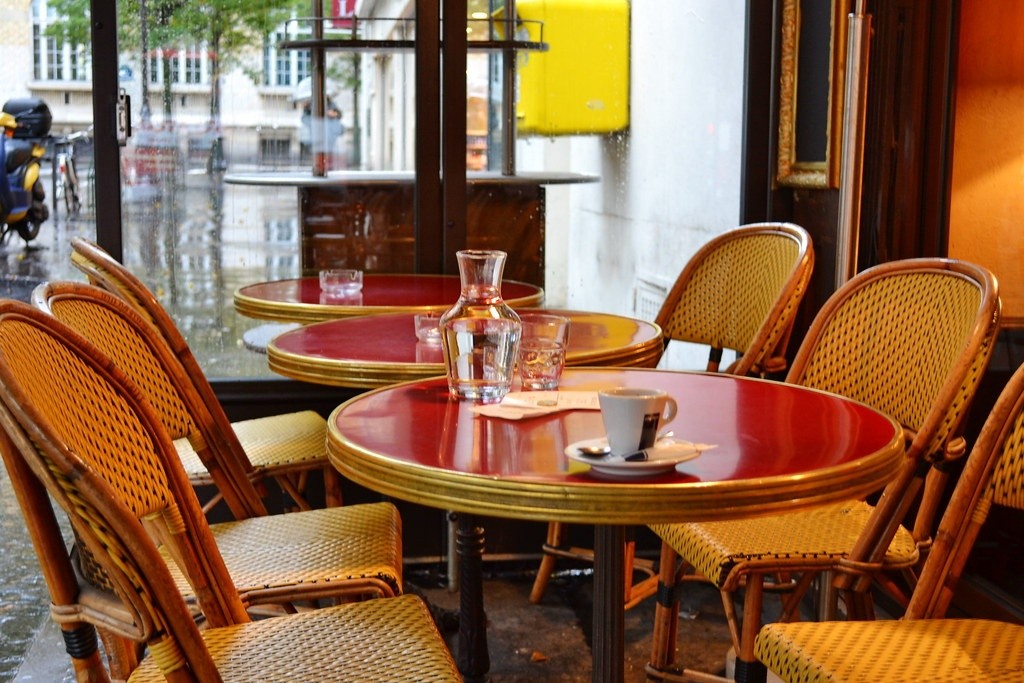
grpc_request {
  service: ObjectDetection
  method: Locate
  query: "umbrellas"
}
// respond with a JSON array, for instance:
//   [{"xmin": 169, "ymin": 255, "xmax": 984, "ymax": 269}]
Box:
[{"xmin": 286, "ymin": 75, "xmax": 345, "ymax": 102}]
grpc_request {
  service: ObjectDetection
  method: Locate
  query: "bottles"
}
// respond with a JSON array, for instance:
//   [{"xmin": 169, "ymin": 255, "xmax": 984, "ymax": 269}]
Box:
[{"xmin": 439, "ymin": 250, "xmax": 523, "ymax": 405}]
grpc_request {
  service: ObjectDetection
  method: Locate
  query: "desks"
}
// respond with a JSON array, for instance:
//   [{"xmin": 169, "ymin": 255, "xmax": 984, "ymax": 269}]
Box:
[
  {"xmin": 325, "ymin": 366, "xmax": 902, "ymax": 683},
  {"xmin": 233, "ymin": 274, "xmax": 545, "ymax": 593},
  {"xmin": 267, "ymin": 308, "xmax": 664, "ymax": 683},
  {"xmin": 224, "ymin": 172, "xmax": 600, "ymax": 291}
]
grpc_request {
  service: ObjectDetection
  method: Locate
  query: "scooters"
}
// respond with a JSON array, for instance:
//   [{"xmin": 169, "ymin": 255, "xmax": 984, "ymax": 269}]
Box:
[{"xmin": 0, "ymin": 97, "xmax": 52, "ymax": 251}]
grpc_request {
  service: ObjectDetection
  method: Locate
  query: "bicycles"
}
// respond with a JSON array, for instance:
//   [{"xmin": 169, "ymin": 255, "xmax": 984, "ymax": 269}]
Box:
[{"xmin": 53, "ymin": 129, "xmax": 90, "ymax": 214}]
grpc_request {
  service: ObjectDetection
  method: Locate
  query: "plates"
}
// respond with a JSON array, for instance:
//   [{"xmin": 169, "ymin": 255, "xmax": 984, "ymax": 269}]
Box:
[{"xmin": 563, "ymin": 438, "xmax": 702, "ymax": 475}]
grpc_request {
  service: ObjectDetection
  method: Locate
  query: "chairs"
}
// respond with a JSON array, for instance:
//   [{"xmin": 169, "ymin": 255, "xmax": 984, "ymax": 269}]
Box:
[
  {"xmin": 530, "ymin": 222, "xmax": 1024, "ymax": 683},
  {"xmin": 0, "ymin": 237, "xmax": 466, "ymax": 683}
]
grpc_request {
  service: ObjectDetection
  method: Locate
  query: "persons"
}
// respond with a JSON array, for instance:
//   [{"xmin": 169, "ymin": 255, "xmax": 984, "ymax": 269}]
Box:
[{"xmin": 302, "ymin": 95, "xmax": 342, "ymax": 171}]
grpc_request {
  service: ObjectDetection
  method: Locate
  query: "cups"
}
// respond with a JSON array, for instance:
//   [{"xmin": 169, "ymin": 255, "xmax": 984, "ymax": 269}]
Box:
[
  {"xmin": 597, "ymin": 388, "xmax": 678, "ymax": 457},
  {"xmin": 516, "ymin": 314, "xmax": 570, "ymax": 390}
]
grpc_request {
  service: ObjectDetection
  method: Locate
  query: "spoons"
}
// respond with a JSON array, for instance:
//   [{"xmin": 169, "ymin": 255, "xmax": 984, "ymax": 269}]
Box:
[{"xmin": 579, "ymin": 428, "xmax": 674, "ymax": 458}]
